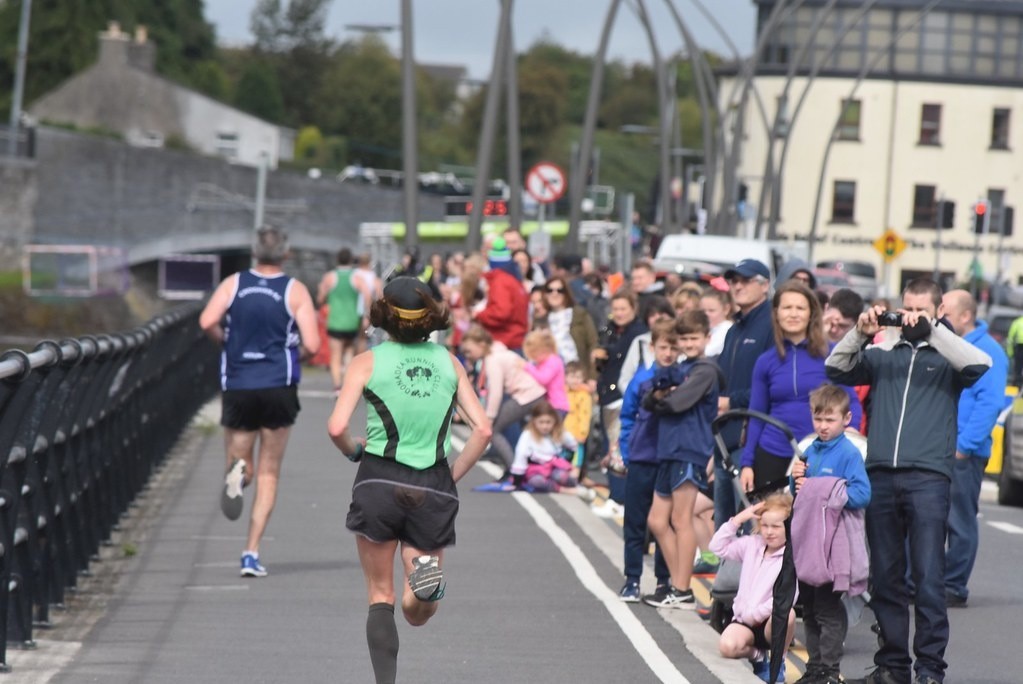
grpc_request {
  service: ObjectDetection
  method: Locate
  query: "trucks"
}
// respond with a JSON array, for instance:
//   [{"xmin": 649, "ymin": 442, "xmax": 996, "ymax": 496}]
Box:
[{"xmin": 653, "ymin": 235, "xmax": 814, "ymax": 301}]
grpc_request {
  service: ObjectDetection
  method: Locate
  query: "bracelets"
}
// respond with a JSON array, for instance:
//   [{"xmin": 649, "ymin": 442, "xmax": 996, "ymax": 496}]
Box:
[{"xmin": 341, "ymin": 438, "xmax": 363, "ymax": 462}]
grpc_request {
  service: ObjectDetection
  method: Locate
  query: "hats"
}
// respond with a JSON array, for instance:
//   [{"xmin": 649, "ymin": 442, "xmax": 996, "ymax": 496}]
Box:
[
  {"xmin": 722, "ymin": 258, "xmax": 770, "ymax": 281},
  {"xmin": 382, "ymin": 277, "xmax": 435, "ymax": 320}
]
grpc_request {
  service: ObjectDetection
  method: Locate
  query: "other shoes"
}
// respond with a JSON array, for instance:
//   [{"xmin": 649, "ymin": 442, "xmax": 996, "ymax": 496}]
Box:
[
  {"xmin": 747, "ymin": 649, "xmax": 786, "ymax": 684},
  {"xmin": 575, "ymin": 486, "xmax": 596, "ymax": 502}
]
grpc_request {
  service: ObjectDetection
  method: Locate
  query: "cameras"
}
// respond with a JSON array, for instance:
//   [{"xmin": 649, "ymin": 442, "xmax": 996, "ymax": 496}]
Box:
[{"xmin": 877, "ymin": 311, "xmax": 902, "ymax": 327}]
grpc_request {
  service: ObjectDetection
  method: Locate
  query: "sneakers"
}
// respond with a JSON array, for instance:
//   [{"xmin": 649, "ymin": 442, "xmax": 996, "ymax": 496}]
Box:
[
  {"xmin": 220, "ymin": 455, "xmax": 248, "ymax": 521},
  {"xmin": 591, "ymin": 499, "xmax": 625, "ymax": 519},
  {"xmin": 793, "ymin": 662, "xmax": 840, "ymax": 684},
  {"xmin": 642, "ymin": 584, "xmax": 698, "ymax": 611},
  {"xmin": 842, "ymin": 664, "xmax": 911, "ymax": 684},
  {"xmin": 240, "ymin": 550, "xmax": 268, "ymax": 578},
  {"xmin": 915, "ymin": 675, "xmax": 941, "ymax": 684},
  {"xmin": 407, "ymin": 553, "xmax": 447, "ymax": 601},
  {"xmin": 617, "ymin": 582, "xmax": 641, "ymax": 603},
  {"xmin": 689, "ymin": 551, "xmax": 722, "ymax": 578}
]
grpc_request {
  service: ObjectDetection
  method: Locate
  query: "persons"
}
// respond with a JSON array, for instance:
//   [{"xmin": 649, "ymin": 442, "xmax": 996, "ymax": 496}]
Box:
[
  {"xmin": 319, "ymin": 227, "xmax": 901, "ymax": 610},
  {"xmin": 823, "ymin": 277, "xmax": 994, "ymax": 684},
  {"xmin": 903, "ymin": 291, "xmax": 1009, "ymax": 609},
  {"xmin": 325, "ymin": 276, "xmax": 495, "ymax": 684},
  {"xmin": 195, "ymin": 224, "xmax": 323, "ymax": 578},
  {"xmin": 789, "ymin": 386, "xmax": 873, "ymax": 684},
  {"xmin": 708, "ymin": 494, "xmax": 798, "ymax": 684}
]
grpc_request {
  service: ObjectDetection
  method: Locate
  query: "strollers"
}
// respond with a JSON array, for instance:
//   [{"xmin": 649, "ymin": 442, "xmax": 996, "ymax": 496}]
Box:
[{"xmin": 711, "ymin": 409, "xmax": 868, "ymax": 634}]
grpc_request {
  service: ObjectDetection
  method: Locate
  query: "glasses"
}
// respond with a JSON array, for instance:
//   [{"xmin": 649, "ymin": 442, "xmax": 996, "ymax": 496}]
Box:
[
  {"xmin": 794, "ymin": 273, "xmax": 813, "ymax": 283},
  {"xmin": 544, "ymin": 285, "xmax": 569, "ymax": 294}
]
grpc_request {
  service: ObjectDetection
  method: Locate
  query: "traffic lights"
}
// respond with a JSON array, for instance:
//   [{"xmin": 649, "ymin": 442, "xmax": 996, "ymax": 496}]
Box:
[{"xmin": 972, "ymin": 200, "xmax": 991, "ymax": 235}]
